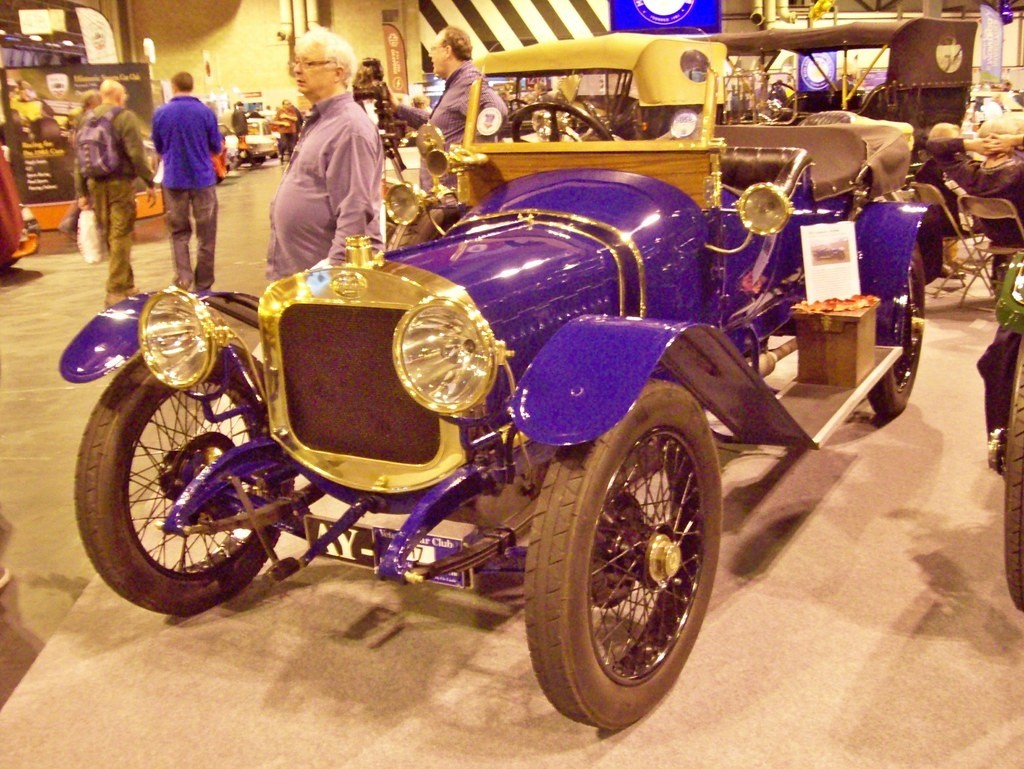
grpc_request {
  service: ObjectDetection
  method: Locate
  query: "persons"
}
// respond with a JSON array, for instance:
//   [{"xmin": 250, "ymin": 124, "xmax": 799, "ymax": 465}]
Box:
[
  {"xmin": 0, "ymin": 109, "xmax": 34, "ymax": 146},
  {"xmin": 974, "ymin": 79, "xmax": 990, "ymax": 91},
  {"xmin": 58, "ymin": 90, "xmax": 99, "ymax": 241},
  {"xmin": 1003, "ymin": 83, "xmax": 1012, "ymax": 92},
  {"xmin": 352, "ymin": 58, "xmax": 383, "ymax": 129},
  {"xmin": 726, "ymin": 84, "xmax": 755, "ymax": 111},
  {"xmin": 383, "ymin": 26, "xmax": 509, "ymax": 192},
  {"xmin": 75, "ymin": 79, "xmax": 155, "ymax": 309},
  {"xmin": 231, "ymin": 101, "xmax": 247, "ymax": 136},
  {"xmin": 756, "ymin": 80, "xmax": 787, "ymax": 102},
  {"xmin": 151, "ymin": 72, "xmax": 221, "ymax": 294},
  {"xmin": 267, "ymin": 30, "xmax": 383, "ymax": 284},
  {"xmin": 23, "ymin": 105, "xmax": 58, "ymax": 145},
  {"xmin": 913, "ymin": 98, "xmax": 1024, "ymax": 279},
  {"xmin": 247, "ymin": 107, "xmax": 264, "ymax": 118},
  {"xmin": 273, "ymin": 99, "xmax": 297, "ymax": 165}
]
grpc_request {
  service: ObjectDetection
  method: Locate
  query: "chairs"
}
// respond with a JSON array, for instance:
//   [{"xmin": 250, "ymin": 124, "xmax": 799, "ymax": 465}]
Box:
[
  {"xmin": 718, "ymin": 146, "xmax": 810, "ymax": 198},
  {"xmin": 956, "ymin": 194, "xmax": 1024, "ymax": 306},
  {"xmin": 907, "ymin": 182, "xmax": 996, "ymax": 298}
]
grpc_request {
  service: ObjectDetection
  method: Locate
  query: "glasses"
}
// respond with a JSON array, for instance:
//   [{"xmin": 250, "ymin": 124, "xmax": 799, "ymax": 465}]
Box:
[{"xmin": 286, "ymin": 58, "xmax": 339, "ymax": 69}]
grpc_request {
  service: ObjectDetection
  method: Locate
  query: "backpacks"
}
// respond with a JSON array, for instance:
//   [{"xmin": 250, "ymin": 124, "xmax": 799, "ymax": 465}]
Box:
[{"xmin": 73, "ymin": 107, "xmax": 128, "ymax": 179}]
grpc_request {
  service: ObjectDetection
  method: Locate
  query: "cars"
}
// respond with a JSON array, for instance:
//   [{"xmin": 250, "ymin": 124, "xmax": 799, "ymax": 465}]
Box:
[
  {"xmin": 57, "ymin": 26, "xmax": 945, "ymax": 735},
  {"xmin": 226, "ymin": 119, "xmax": 279, "ymax": 159}
]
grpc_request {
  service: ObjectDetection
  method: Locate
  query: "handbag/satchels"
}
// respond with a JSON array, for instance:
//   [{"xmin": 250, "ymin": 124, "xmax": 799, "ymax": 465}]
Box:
[{"xmin": 75, "ymin": 210, "xmax": 104, "ymax": 264}]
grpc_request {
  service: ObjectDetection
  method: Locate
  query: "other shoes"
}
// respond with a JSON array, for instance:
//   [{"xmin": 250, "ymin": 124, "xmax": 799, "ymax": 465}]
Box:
[
  {"xmin": 942, "ymin": 258, "xmax": 980, "ymax": 279},
  {"xmin": 59, "ymin": 215, "xmax": 78, "ymax": 240}
]
